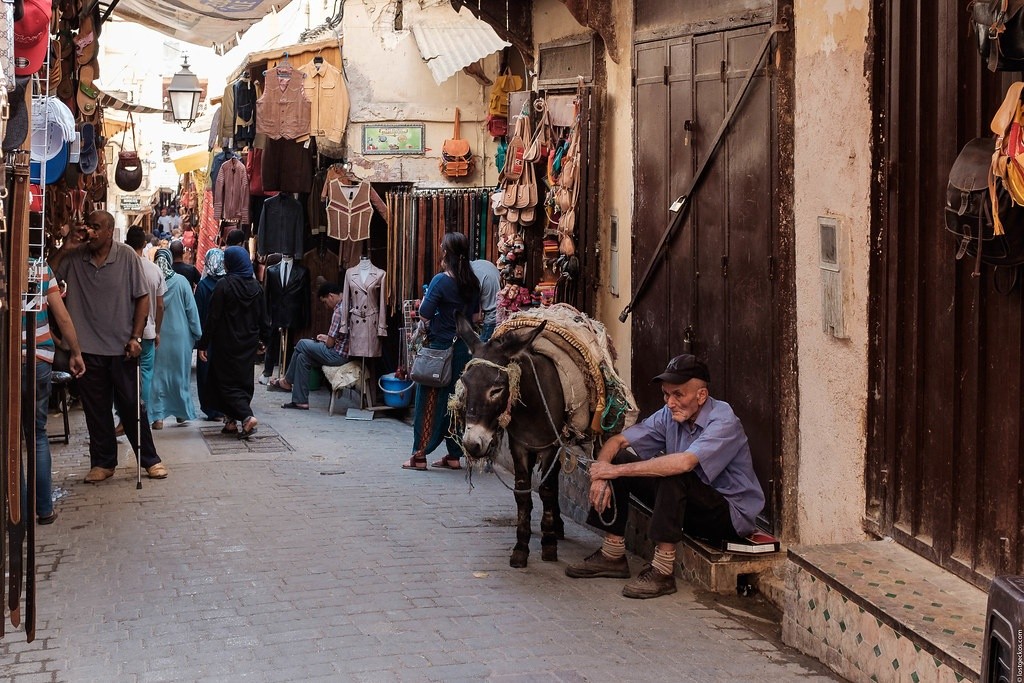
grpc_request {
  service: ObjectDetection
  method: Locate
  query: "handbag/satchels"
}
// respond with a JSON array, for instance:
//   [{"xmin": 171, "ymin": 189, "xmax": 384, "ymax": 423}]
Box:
[
  {"xmin": 410, "ymin": 334, "xmax": 454, "ymax": 387},
  {"xmin": 966, "ymin": 0, "xmax": 1024, "ymax": 73},
  {"xmin": 496, "ymin": 112, "xmax": 582, "ymax": 256},
  {"xmin": 987, "ymin": 80, "xmax": 1024, "ymax": 235},
  {"xmin": 116, "ymin": 110, "xmax": 142, "ymax": 192},
  {"xmin": 0, "ymin": 0, "xmax": 14, "ymax": 152},
  {"xmin": 944, "ymin": 128, "xmax": 1023, "ymax": 296}
]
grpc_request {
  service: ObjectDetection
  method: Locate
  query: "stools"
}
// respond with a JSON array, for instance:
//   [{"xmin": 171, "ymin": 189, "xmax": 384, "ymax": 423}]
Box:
[
  {"xmin": 47, "ymin": 370, "xmax": 73, "ymax": 446},
  {"xmin": 322, "ymin": 360, "xmax": 373, "ymax": 417}
]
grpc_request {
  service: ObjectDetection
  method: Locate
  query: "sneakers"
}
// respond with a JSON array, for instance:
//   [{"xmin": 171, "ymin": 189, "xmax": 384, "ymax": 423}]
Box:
[
  {"xmin": 84, "ymin": 466, "xmax": 116, "ymax": 481},
  {"xmin": 623, "ymin": 560, "xmax": 677, "ymax": 599},
  {"xmin": 565, "ymin": 545, "xmax": 631, "ymax": 578},
  {"xmin": 147, "ymin": 462, "xmax": 167, "ymax": 478}
]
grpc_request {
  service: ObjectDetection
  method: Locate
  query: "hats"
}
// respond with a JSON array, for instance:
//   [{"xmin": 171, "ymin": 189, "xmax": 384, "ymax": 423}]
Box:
[
  {"xmin": 652, "ymin": 355, "xmax": 711, "ymax": 387},
  {"xmin": 3, "ymin": 0, "xmax": 75, "ymax": 185}
]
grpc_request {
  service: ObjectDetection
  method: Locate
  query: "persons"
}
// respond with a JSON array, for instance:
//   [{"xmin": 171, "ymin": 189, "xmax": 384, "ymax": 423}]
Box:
[
  {"xmin": 339, "ymin": 256, "xmax": 389, "ymax": 358},
  {"xmin": 47, "ymin": 209, "xmax": 169, "ymax": 481},
  {"xmin": 121, "ymin": 209, "xmax": 358, "ymax": 440},
  {"xmin": 21, "ymin": 212, "xmax": 85, "ymax": 524},
  {"xmin": 469, "ymin": 256, "xmax": 501, "ymax": 340},
  {"xmin": 565, "ymin": 353, "xmax": 766, "ymax": 599},
  {"xmin": 402, "ymin": 234, "xmax": 482, "ymax": 470}
]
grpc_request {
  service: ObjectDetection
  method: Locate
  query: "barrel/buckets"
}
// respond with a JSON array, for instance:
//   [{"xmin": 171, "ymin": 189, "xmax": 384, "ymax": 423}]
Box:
[{"xmin": 378, "ymin": 374, "xmax": 415, "ymax": 408}]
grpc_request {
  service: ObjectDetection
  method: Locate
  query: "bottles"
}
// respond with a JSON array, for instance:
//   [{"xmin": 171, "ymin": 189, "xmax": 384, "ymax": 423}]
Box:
[
  {"xmin": 423, "ymin": 284, "xmax": 440, "ymax": 316},
  {"xmin": 78, "ymin": 227, "xmax": 96, "ymax": 242}
]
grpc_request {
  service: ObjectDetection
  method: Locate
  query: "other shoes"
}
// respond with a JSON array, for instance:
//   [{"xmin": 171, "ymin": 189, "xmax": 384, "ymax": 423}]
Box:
[
  {"xmin": 115, "ymin": 420, "xmax": 125, "ymax": 437},
  {"xmin": 177, "ymin": 416, "xmax": 184, "ymax": 423},
  {"xmin": 152, "ymin": 420, "xmax": 164, "ymax": 429}
]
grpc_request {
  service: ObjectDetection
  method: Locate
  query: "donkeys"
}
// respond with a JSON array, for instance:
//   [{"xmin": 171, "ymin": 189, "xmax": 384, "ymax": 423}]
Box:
[{"xmin": 453, "ymin": 310, "xmax": 608, "ymax": 568}]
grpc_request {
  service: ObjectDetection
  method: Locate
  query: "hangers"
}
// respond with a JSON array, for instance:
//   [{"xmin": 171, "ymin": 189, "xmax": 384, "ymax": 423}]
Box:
[
  {"xmin": 327, "ymin": 161, "xmax": 372, "ymax": 188},
  {"xmin": 262, "ymin": 51, "xmax": 307, "ymax": 78},
  {"xmin": 314, "ymin": 46, "xmax": 324, "ymax": 64},
  {"xmin": 232, "ymin": 152, "xmax": 238, "ymax": 159},
  {"xmin": 243, "ymin": 70, "xmax": 250, "ymax": 78}
]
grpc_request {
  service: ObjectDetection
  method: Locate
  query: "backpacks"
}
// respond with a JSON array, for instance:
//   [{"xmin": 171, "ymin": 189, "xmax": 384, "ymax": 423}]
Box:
[
  {"xmin": 439, "ymin": 107, "xmax": 475, "ymax": 181},
  {"xmin": 488, "ymin": 65, "xmax": 522, "ymax": 136}
]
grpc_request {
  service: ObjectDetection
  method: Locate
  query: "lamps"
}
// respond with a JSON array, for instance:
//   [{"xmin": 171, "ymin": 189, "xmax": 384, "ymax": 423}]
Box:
[{"xmin": 165, "ymin": 54, "xmax": 204, "ymax": 133}]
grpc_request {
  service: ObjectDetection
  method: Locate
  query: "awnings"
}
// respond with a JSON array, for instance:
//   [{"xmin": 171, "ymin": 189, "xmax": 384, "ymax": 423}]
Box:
[{"xmin": 92, "ymin": 0, "xmax": 294, "ymax": 58}]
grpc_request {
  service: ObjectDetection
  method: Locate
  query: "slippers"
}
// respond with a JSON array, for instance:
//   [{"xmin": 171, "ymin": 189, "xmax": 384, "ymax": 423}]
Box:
[
  {"xmin": 402, "ymin": 457, "xmax": 429, "ymax": 470},
  {"xmin": 270, "ymin": 378, "xmax": 293, "ymax": 392},
  {"xmin": 48, "ymin": 0, "xmax": 109, "ymax": 242},
  {"xmin": 237, "ymin": 426, "xmax": 256, "ymax": 439},
  {"xmin": 36, "ymin": 507, "xmax": 57, "ymax": 526},
  {"xmin": 221, "ymin": 425, "xmax": 238, "ymax": 433},
  {"xmin": 280, "ymin": 401, "xmax": 309, "ymax": 410},
  {"xmin": 432, "ymin": 456, "xmax": 462, "ymax": 469}
]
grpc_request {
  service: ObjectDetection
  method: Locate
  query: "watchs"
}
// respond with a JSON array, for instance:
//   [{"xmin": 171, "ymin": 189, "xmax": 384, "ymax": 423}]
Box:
[{"xmin": 130, "ymin": 335, "xmax": 142, "ymax": 344}]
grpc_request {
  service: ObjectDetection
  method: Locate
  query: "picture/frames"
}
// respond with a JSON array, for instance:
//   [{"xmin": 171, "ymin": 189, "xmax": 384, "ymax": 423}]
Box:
[{"xmin": 360, "ymin": 123, "xmax": 426, "ymax": 156}]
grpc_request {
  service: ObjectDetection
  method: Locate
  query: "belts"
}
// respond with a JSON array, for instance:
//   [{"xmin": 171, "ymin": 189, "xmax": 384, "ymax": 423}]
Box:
[{"xmin": 385, "ymin": 185, "xmax": 499, "ymax": 319}]
